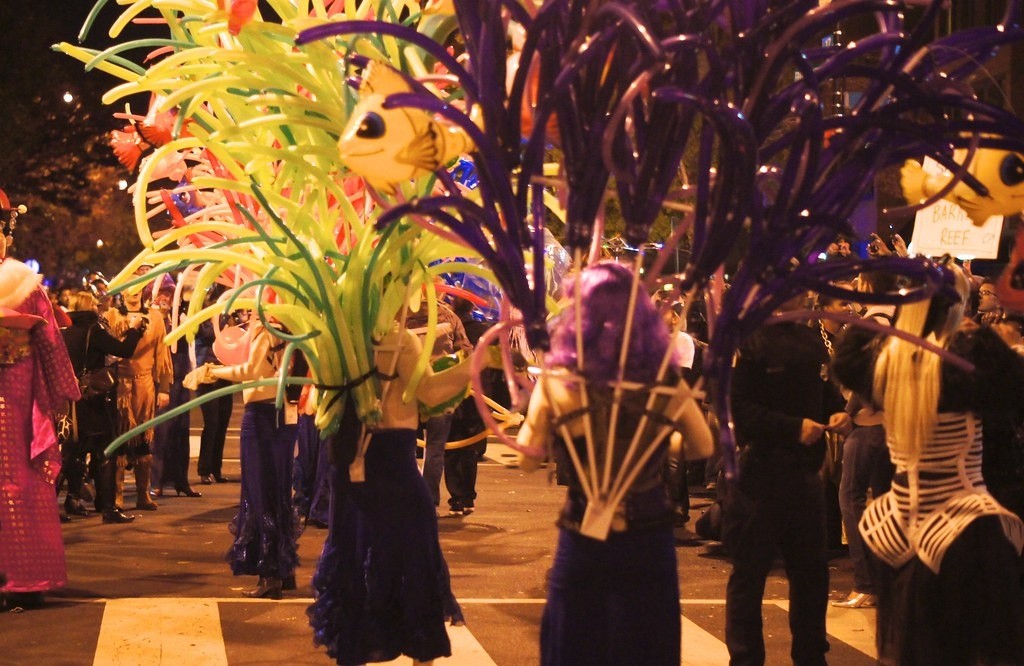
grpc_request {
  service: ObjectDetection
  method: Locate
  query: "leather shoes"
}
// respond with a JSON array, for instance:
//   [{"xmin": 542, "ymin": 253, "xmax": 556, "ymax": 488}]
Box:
[
  {"xmin": 201, "ymin": 474, "xmax": 211, "ymax": 484},
  {"xmin": 215, "ymin": 473, "xmax": 229, "ymax": 483}
]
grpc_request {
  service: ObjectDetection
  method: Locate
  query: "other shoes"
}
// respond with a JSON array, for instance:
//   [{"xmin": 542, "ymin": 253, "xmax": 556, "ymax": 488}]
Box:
[
  {"xmin": 449, "ymin": 510, "xmax": 463, "ymax": 517},
  {"xmin": 464, "ymin": 507, "xmax": 474, "ymax": 513},
  {"xmin": 832, "ymin": 593, "xmax": 874, "ymax": 608}
]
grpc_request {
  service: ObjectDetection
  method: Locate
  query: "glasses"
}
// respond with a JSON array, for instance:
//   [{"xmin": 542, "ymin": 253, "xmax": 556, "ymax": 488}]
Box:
[{"xmin": 979, "ymin": 290, "xmax": 999, "ymax": 299}]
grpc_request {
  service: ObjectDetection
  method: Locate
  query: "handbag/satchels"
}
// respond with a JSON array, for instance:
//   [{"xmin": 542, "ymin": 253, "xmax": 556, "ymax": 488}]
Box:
[{"xmin": 78, "ymin": 322, "xmax": 114, "ymax": 402}]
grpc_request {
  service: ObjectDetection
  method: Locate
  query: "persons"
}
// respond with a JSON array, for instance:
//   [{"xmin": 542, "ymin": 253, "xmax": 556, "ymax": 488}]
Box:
[{"xmin": 0, "ymin": 189, "xmax": 1024, "ymax": 666}]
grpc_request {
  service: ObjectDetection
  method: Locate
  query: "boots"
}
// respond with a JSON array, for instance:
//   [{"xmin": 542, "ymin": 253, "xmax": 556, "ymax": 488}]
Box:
[
  {"xmin": 94, "ymin": 461, "xmax": 123, "ymax": 513},
  {"xmin": 100, "ymin": 460, "xmax": 135, "ymax": 522},
  {"xmin": 64, "ymin": 457, "xmax": 90, "ymax": 517},
  {"xmin": 134, "ymin": 454, "xmax": 158, "ymax": 510}
]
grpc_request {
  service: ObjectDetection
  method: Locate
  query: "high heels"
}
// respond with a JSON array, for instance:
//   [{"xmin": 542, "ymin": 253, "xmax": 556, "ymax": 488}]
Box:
[
  {"xmin": 283, "ymin": 571, "xmax": 297, "ymax": 590},
  {"xmin": 150, "ymin": 486, "xmax": 162, "ymax": 496},
  {"xmin": 243, "ymin": 574, "xmax": 283, "ymax": 600},
  {"xmin": 175, "ymin": 484, "xmax": 202, "ymax": 497}
]
